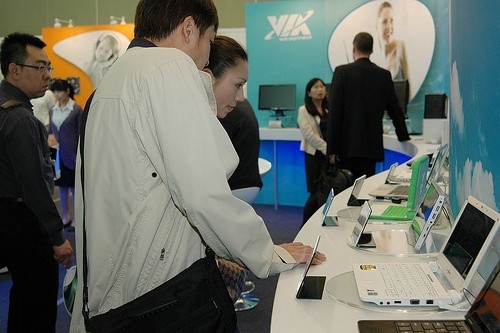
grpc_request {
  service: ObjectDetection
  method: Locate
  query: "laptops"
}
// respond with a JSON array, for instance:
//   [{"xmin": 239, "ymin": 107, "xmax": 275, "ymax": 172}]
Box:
[
  {"xmin": 388, "ymin": 144, "xmax": 448, "ymax": 187},
  {"xmin": 358, "ymin": 261, "xmax": 500, "ymax": 333},
  {"xmin": 352, "ymin": 195, "xmax": 500, "ymax": 307},
  {"xmin": 363, "ymin": 155, "xmax": 430, "ymax": 220}
]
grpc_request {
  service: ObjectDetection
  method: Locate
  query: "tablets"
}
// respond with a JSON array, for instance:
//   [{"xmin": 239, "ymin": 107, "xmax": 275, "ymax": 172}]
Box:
[{"xmin": 409, "ymin": 183, "xmax": 444, "ymax": 252}]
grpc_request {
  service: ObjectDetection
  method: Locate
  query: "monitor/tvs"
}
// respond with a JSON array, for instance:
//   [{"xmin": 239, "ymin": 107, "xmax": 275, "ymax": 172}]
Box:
[
  {"xmin": 424, "ymin": 94, "xmax": 448, "ymax": 119},
  {"xmin": 382, "ymin": 80, "xmax": 409, "ymax": 119},
  {"xmin": 259, "ymin": 84, "xmax": 297, "ymax": 117}
]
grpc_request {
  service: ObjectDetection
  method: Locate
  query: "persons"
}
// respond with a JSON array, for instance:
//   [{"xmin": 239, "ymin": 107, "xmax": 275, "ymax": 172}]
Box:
[
  {"xmin": 297, "ymin": 78, "xmax": 327, "ymax": 232},
  {"xmin": 216, "ymin": 98, "xmax": 263, "ymax": 205},
  {"xmin": 370, "ymin": 1, "xmax": 412, "ymax": 102},
  {"xmin": 69, "ymin": 0, "xmax": 327, "ymax": 333},
  {"xmin": 0, "ymin": 31, "xmax": 73, "ymax": 333},
  {"xmin": 76, "ymin": 34, "xmax": 118, "ymax": 91},
  {"xmin": 328, "ymin": 31, "xmax": 412, "ymax": 184},
  {"xmin": 30, "ymin": 86, "xmax": 57, "ymax": 185},
  {"xmin": 201, "ymin": 35, "xmax": 248, "ymax": 119},
  {"xmin": 47, "ymin": 78, "xmax": 83, "ymax": 231}
]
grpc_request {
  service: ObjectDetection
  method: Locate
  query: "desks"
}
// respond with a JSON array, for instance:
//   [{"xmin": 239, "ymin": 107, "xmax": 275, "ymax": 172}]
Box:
[{"xmin": 257, "ymin": 126, "xmax": 468, "ymax": 332}]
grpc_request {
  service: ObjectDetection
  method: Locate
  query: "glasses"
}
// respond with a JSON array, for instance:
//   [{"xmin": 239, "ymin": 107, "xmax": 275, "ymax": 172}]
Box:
[{"xmin": 16, "ymin": 64, "xmax": 52, "ymax": 74}]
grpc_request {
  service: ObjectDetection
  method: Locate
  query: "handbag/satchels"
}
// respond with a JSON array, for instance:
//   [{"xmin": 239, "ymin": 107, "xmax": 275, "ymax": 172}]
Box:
[
  {"xmin": 323, "ymin": 163, "xmax": 354, "ymax": 200},
  {"xmin": 83, "ymin": 257, "xmax": 238, "ymax": 333},
  {"xmin": 215, "ymin": 256, "xmax": 249, "ymax": 302}
]
